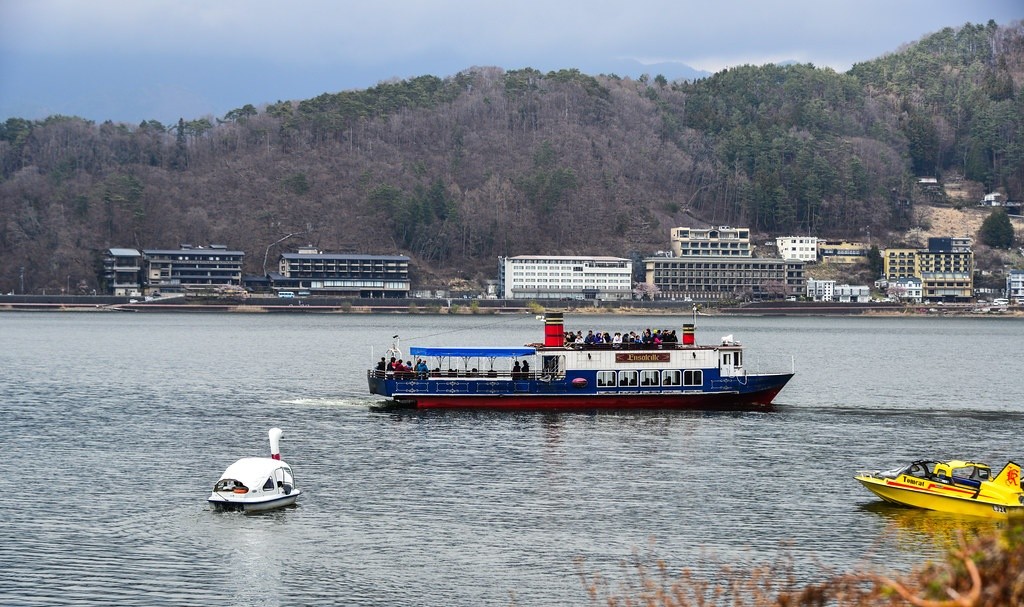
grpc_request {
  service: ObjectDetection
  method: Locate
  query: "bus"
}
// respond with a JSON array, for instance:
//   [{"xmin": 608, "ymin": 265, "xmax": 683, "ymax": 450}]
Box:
[
  {"xmin": 994, "ymin": 299, "xmax": 1009, "ymax": 305},
  {"xmin": 1018, "ymin": 299, "xmax": 1024, "ymax": 305},
  {"xmin": 278, "ymin": 292, "xmax": 294, "ymax": 298},
  {"xmin": 786, "ymin": 296, "xmax": 796, "ymax": 302}
]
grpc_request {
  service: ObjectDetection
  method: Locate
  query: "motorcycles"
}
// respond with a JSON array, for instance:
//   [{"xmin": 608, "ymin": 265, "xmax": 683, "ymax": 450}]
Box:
[{"xmin": 852, "ymin": 458, "xmax": 1024, "ymax": 520}]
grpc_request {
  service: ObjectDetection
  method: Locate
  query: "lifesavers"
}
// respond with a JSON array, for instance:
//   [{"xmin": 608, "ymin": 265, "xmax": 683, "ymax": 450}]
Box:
[{"xmin": 234, "ymin": 487, "xmax": 248, "ymax": 494}]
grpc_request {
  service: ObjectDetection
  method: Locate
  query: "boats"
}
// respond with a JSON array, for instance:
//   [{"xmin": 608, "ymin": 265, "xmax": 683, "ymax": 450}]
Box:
[
  {"xmin": 207, "ymin": 428, "xmax": 301, "ymax": 513},
  {"xmin": 365, "ymin": 312, "xmax": 795, "ymax": 411}
]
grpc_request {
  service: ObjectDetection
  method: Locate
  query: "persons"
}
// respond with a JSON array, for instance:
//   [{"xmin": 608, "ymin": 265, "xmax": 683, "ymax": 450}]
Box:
[
  {"xmin": 222, "ymin": 481, "xmax": 248, "ymax": 491},
  {"xmin": 472, "ymin": 368, "xmax": 478, "ymax": 377},
  {"xmin": 512, "ymin": 361, "xmax": 521, "ymax": 380},
  {"xmin": 433, "ymin": 367, "xmax": 441, "ymax": 377},
  {"xmin": 563, "ymin": 328, "xmax": 678, "ymax": 350},
  {"xmin": 521, "ymin": 360, "xmax": 530, "ymax": 380},
  {"xmin": 598, "ymin": 378, "xmax": 612, "ymax": 386},
  {"xmin": 278, "ymin": 481, "xmax": 282, "ymax": 487},
  {"xmin": 376, "ymin": 357, "xmax": 429, "ymax": 380},
  {"xmin": 663, "ymin": 376, "xmax": 671, "ymax": 385},
  {"xmin": 642, "ymin": 378, "xmax": 653, "ymax": 385},
  {"xmin": 620, "ymin": 377, "xmax": 635, "ymax": 386}
]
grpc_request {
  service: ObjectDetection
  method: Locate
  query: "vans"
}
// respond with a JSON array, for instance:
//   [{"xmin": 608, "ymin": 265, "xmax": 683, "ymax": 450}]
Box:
[
  {"xmin": 994, "ymin": 302, "xmax": 998, "ymax": 306},
  {"xmin": 575, "ymin": 296, "xmax": 585, "ymax": 301}
]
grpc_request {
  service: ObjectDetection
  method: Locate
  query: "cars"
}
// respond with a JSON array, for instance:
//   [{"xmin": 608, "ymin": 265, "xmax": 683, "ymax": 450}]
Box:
[{"xmin": 684, "ymin": 297, "xmax": 692, "ymax": 302}]
